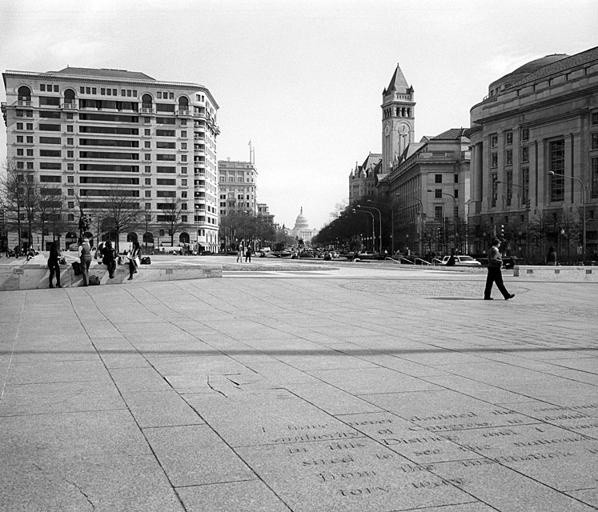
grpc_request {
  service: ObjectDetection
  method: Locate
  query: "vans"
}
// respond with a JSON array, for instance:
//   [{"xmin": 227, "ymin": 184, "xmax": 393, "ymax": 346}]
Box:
[{"xmin": 441, "ymin": 255, "xmax": 481, "ymax": 267}]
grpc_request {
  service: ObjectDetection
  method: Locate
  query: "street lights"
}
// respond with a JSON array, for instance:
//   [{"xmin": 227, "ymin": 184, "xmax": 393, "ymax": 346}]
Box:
[
  {"xmin": 196, "ymin": 205, "xmax": 199, "ymax": 246},
  {"xmin": 395, "ymin": 193, "xmax": 424, "ymax": 254},
  {"xmin": 352, "ymin": 200, "xmax": 394, "ymax": 254},
  {"xmin": 455, "ymin": 232, "xmax": 460, "ymax": 251},
  {"xmin": 242, "ymin": 210, "xmax": 248, "ymax": 241},
  {"xmin": 426, "ymin": 189, "xmax": 457, "ymax": 253},
  {"xmin": 494, "ymin": 180, "xmax": 530, "ymax": 258},
  {"xmin": 559, "ymin": 228, "xmax": 565, "ymax": 256},
  {"xmin": 517, "ymin": 231, "xmax": 523, "ymax": 254},
  {"xmin": 406, "ymin": 234, "xmax": 409, "ymax": 247},
  {"xmin": 546, "ymin": 170, "xmax": 586, "ymax": 259},
  {"xmin": 482, "ymin": 232, "xmax": 487, "ymax": 254}
]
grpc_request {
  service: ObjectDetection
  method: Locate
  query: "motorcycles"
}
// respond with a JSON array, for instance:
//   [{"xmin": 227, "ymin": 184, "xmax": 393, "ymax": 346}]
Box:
[{"xmin": 6, "ymin": 246, "xmax": 20, "ymax": 259}]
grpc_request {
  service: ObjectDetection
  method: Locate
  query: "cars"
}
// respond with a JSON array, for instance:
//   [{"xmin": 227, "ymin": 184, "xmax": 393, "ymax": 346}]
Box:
[
  {"xmin": 256, "ymin": 245, "xmax": 385, "ymax": 261},
  {"xmin": 510, "ymin": 255, "xmax": 526, "ymax": 265},
  {"xmin": 69, "ymin": 243, "xmax": 78, "ymax": 251}
]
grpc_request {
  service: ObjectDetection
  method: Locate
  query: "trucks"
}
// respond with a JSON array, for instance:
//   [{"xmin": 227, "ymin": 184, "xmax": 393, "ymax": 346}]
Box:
[{"xmin": 98, "ymin": 241, "xmax": 134, "ymax": 255}]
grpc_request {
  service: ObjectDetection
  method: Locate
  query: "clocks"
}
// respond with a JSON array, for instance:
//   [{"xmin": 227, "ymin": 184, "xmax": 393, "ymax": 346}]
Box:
[
  {"xmin": 384, "ymin": 121, "xmax": 392, "ymax": 138},
  {"xmin": 398, "ymin": 121, "xmax": 411, "ymax": 136}
]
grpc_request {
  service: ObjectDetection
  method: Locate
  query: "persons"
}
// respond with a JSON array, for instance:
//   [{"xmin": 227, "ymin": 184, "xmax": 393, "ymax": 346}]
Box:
[
  {"xmin": 78, "ymin": 238, "xmax": 92, "ymax": 287},
  {"xmin": 484, "ymin": 239, "xmax": 515, "ymax": 300},
  {"xmin": 94, "ymin": 243, "xmax": 105, "ymax": 264},
  {"xmin": 128, "ymin": 242, "xmax": 142, "ymax": 280},
  {"xmin": 101, "ymin": 240, "xmax": 116, "ymax": 278},
  {"xmin": 48, "ymin": 243, "xmax": 62, "ymax": 288},
  {"xmin": 237, "ymin": 241, "xmax": 243, "ymax": 263},
  {"xmin": 245, "ymin": 243, "xmax": 252, "ymax": 263}
]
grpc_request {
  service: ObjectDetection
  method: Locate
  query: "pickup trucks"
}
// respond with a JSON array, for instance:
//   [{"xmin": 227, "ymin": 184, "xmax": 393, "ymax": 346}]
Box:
[{"xmin": 153, "ymin": 241, "xmax": 183, "ymax": 254}]
